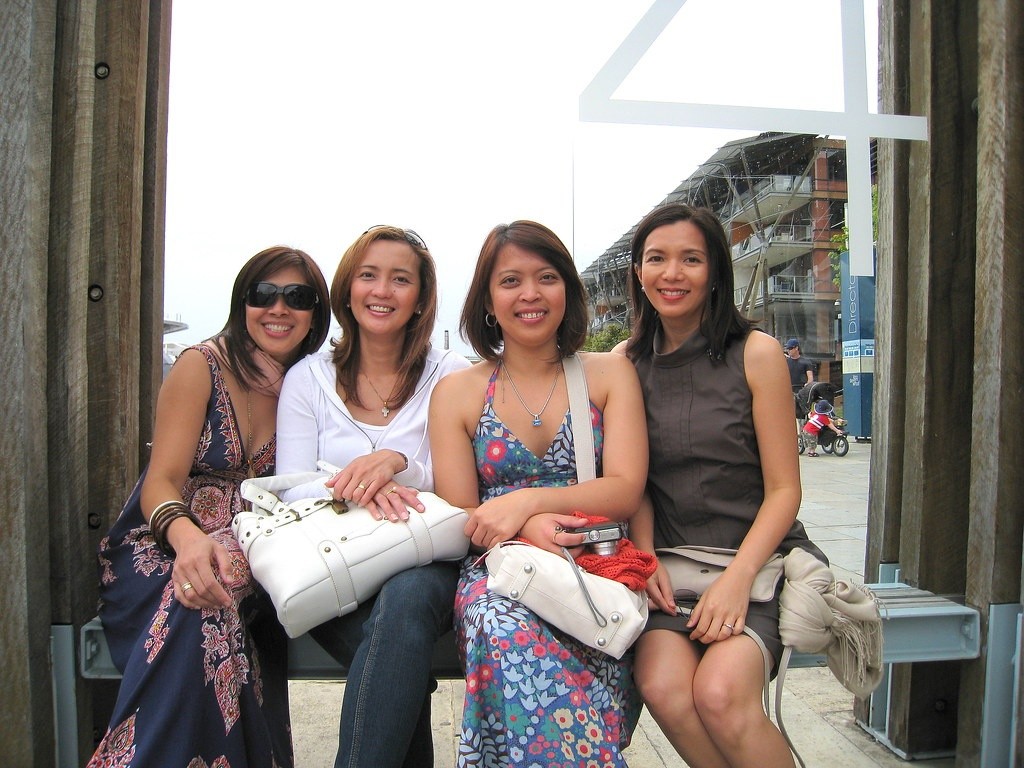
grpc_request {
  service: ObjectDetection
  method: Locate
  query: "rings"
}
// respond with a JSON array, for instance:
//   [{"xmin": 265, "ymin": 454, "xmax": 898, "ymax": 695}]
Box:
[
  {"xmin": 358, "ymin": 485, "xmax": 366, "ymax": 490},
  {"xmin": 723, "ymin": 624, "xmax": 734, "ymax": 630},
  {"xmin": 386, "ymin": 486, "xmax": 397, "ymax": 496},
  {"xmin": 180, "ymin": 581, "xmax": 192, "ymax": 593},
  {"xmin": 553, "ymin": 526, "xmax": 567, "ymax": 544},
  {"xmin": 647, "ymin": 597, "xmax": 651, "ymax": 601}
]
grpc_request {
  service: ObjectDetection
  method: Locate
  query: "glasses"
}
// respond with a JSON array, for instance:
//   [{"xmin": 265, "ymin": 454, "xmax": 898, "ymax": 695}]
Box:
[
  {"xmin": 787, "ymin": 347, "xmax": 795, "ymax": 350},
  {"xmin": 242, "ymin": 280, "xmax": 320, "ymax": 311},
  {"xmin": 362, "ymin": 224, "xmax": 427, "ymax": 251}
]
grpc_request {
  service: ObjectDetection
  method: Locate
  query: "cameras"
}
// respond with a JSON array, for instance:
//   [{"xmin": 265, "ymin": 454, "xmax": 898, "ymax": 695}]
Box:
[{"xmin": 565, "ymin": 521, "xmax": 622, "ymax": 556}]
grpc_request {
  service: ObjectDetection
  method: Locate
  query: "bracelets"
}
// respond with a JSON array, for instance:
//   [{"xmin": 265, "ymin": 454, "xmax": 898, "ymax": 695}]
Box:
[
  {"xmin": 148, "ymin": 501, "xmax": 203, "ymax": 558},
  {"xmin": 516, "ymin": 530, "xmax": 520, "ymax": 537},
  {"xmin": 395, "ymin": 451, "xmax": 408, "ymax": 469}
]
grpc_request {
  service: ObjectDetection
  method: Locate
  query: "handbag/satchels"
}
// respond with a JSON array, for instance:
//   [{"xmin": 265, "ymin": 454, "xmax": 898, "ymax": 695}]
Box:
[
  {"xmin": 655, "ymin": 544, "xmax": 784, "ymax": 602},
  {"xmin": 470, "ymin": 541, "xmax": 649, "ymax": 661},
  {"xmin": 230, "ymin": 460, "xmax": 471, "ymax": 639}
]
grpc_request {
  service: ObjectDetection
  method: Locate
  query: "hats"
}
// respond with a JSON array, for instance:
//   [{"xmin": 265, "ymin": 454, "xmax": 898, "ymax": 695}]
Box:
[
  {"xmin": 814, "ymin": 400, "xmax": 834, "ymax": 414},
  {"xmin": 784, "ymin": 339, "xmax": 799, "ymax": 348}
]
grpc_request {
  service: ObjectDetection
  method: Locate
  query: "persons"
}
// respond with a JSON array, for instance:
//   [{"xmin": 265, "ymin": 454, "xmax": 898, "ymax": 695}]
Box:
[
  {"xmin": 784, "ymin": 338, "xmax": 813, "ymax": 448},
  {"xmin": 803, "ymin": 400, "xmax": 848, "ymax": 457},
  {"xmin": 276, "ymin": 226, "xmax": 474, "ymax": 768},
  {"xmin": 610, "ymin": 205, "xmax": 829, "ymax": 768},
  {"xmin": 85, "ymin": 246, "xmax": 331, "ymax": 768},
  {"xmin": 429, "ymin": 220, "xmax": 649, "ymax": 768}
]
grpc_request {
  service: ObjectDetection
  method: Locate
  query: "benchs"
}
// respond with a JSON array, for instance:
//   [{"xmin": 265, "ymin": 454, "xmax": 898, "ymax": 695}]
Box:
[{"xmin": 79, "ymin": 582, "xmax": 982, "ymax": 680}]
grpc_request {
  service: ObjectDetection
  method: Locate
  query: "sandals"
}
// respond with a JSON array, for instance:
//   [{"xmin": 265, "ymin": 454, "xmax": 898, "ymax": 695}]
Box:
[{"xmin": 808, "ymin": 452, "xmax": 819, "ymax": 457}]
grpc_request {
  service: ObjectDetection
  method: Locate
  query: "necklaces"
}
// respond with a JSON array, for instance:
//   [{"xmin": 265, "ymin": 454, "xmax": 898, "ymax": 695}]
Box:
[
  {"xmin": 500, "ymin": 351, "xmax": 561, "ymax": 426},
  {"xmin": 360, "ymin": 367, "xmax": 400, "ymax": 417},
  {"xmin": 248, "ymin": 387, "xmax": 276, "ymax": 479}
]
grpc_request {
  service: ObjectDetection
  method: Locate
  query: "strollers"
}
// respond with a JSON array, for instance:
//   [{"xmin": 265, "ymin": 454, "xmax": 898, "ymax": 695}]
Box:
[{"xmin": 793, "ymin": 380, "xmax": 849, "ymax": 458}]
